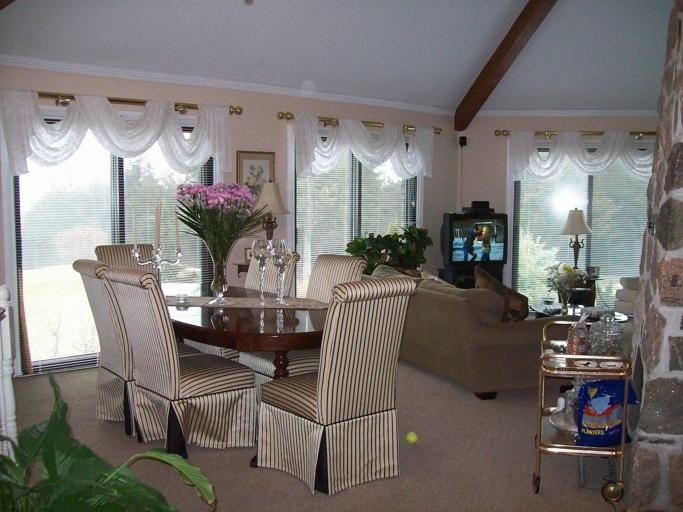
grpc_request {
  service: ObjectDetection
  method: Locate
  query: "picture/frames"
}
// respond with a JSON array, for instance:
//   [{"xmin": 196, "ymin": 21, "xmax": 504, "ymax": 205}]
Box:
[{"xmin": 236, "ymin": 150, "xmax": 276, "ymax": 192}]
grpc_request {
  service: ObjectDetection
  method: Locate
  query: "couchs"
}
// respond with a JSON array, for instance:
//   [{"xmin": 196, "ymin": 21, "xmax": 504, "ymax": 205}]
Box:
[{"xmin": 362, "ymin": 264, "xmax": 589, "ymax": 399}]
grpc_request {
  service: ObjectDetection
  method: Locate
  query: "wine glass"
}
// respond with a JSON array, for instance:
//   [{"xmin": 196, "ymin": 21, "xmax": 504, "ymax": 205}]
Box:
[{"xmin": 251, "ymin": 238, "xmax": 293, "ymax": 307}]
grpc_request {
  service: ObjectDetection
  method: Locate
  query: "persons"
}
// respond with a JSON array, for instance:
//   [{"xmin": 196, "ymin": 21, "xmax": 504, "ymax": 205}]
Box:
[{"xmin": 462, "ymin": 225, "xmax": 491, "ymax": 262}]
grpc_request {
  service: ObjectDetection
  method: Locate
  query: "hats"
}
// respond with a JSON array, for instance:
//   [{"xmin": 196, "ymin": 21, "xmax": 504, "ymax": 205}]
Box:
[{"xmin": 472, "ymin": 224, "xmax": 479, "ymax": 230}]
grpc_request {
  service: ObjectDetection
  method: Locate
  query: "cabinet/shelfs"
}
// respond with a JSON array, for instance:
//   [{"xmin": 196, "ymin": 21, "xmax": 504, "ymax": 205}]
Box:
[{"xmin": 558, "ymin": 278, "xmax": 604, "ymax": 307}]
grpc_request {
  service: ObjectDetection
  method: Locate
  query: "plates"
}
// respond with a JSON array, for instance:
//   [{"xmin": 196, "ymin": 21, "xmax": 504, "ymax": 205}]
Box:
[
  {"xmin": 599, "ymin": 361, "xmax": 624, "ymax": 370},
  {"xmin": 574, "ymin": 361, "xmax": 597, "ymax": 370}
]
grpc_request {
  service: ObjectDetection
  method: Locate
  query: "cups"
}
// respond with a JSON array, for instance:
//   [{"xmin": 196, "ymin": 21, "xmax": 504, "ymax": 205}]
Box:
[
  {"xmin": 176, "ymin": 293, "xmax": 188, "ymax": 303},
  {"xmin": 589, "ymin": 314, "xmax": 624, "ymax": 357}
]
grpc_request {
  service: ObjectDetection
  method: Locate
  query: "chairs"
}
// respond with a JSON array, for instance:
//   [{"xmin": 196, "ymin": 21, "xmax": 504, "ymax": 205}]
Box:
[
  {"xmin": 256, "ymin": 277, "xmax": 417, "ymax": 495},
  {"xmin": 183, "ymin": 252, "xmax": 300, "ymax": 359},
  {"xmin": 95, "ymin": 244, "xmax": 159, "ymax": 283},
  {"xmin": 73, "ymin": 259, "xmax": 201, "ymax": 436},
  {"xmin": 105, "ymin": 267, "xmax": 255, "ymax": 459},
  {"xmin": 238, "ymin": 255, "xmax": 368, "ymax": 395}
]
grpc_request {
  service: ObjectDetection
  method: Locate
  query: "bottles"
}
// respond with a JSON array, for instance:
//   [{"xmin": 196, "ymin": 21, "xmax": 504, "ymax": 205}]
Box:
[
  {"xmin": 577, "ymin": 323, "xmax": 589, "ymax": 355},
  {"xmin": 542, "ymin": 290, "xmax": 555, "ymax": 312},
  {"xmin": 565, "ymin": 326, "xmax": 576, "ymax": 355}
]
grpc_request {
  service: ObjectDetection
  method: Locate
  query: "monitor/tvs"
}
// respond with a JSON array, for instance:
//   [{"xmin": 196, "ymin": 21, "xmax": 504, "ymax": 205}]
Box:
[{"xmin": 441, "ymin": 214, "xmax": 507, "ymax": 267}]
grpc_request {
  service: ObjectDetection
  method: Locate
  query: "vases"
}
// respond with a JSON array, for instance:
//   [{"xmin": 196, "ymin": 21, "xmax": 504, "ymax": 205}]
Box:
[
  {"xmin": 202, "ymin": 240, "xmax": 238, "ymax": 306},
  {"xmin": 559, "ymin": 292, "xmax": 571, "ymax": 315}
]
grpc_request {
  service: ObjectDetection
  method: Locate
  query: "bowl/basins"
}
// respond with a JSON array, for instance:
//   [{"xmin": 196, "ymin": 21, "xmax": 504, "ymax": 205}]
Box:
[{"xmin": 548, "ymin": 409, "xmax": 580, "ymax": 437}]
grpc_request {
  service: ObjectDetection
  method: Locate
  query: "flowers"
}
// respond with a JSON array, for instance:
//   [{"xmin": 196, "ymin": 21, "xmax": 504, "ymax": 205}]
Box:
[
  {"xmin": 546, "ymin": 263, "xmax": 587, "ymax": 308},
  {"xmin": 176, "ymin": 181, "xmax": 256, "ymax": 240}
]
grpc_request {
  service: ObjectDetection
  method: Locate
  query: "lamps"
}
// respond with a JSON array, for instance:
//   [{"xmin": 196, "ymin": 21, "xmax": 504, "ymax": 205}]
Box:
[
  {"xmin": 256, "ymin": 183, "xmax": 291, "ymax": 240},
  {"xmin": 561, "ymin": 208, "xmax": 592, "ymax": 269}
]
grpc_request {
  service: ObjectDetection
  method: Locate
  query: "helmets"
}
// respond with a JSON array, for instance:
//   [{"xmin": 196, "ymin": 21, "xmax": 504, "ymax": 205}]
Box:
[{"xmin": 481, "ymin": 226, "xmax": 491, "ymax": 234}]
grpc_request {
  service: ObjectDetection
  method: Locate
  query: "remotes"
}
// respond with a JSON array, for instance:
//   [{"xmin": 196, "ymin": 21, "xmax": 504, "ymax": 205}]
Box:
[{"xmin": 548, "ymin": 308, "xmax": 561, "ymax": 315}]
